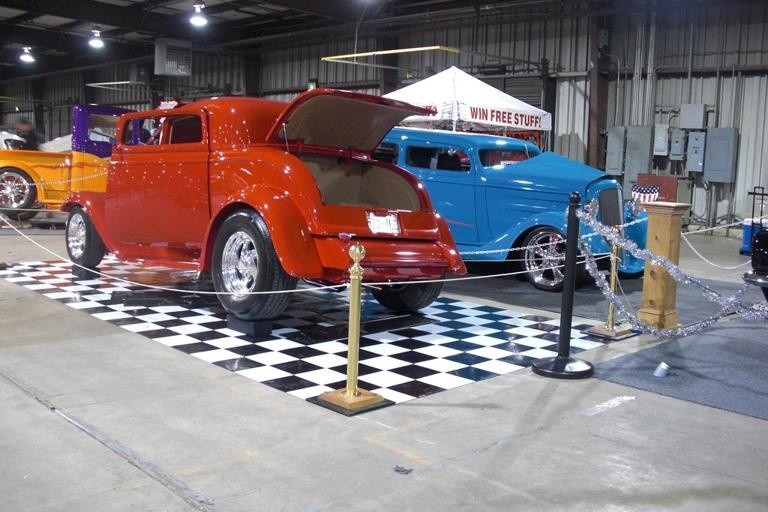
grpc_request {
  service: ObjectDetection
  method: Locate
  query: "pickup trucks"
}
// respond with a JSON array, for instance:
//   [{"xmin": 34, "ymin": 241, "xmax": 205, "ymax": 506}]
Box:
[{"xmin": 0, "ymin": 104, "xmax": 148, "ymax": 220}]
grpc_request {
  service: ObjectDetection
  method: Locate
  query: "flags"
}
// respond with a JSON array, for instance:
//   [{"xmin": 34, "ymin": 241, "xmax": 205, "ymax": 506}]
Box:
[{"xmin": 628, "ymin": 182, "xmax": 661, "ymax": 214}]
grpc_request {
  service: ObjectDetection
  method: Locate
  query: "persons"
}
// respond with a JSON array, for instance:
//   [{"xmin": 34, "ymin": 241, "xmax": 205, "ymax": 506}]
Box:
[
  {"xmin": 10, "ymin": 116, "xmax": 39, "ymax": 151},
  {"xmin": 125, "ymin": 101, "xmax": 182, "ymax": 144},
  {"xmin": 407, "ymin": 146, "xmax": 461, "ymax": 171}
]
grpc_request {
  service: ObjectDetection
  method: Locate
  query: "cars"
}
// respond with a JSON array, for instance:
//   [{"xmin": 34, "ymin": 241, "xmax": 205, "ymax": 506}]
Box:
[
  {"xmin": 60, "ymin": 87, "xmax": 467, "ymax": 325},
  {"xmin": 376, "ymin": 129, "xmax": 650, "ymax": 291}
]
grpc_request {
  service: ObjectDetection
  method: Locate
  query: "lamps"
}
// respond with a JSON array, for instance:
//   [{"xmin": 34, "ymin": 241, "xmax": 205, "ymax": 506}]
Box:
[
  {"xmin": 189, "ymin": 0, "xmax": 208, "ymax": 27},
  {"xmin": 18, "ymin": 45, "xmax": 34, "ymax": 63},
  {"xmin": 88, "ymin": 27, "xmax": 104, "ymax": 47}
]
